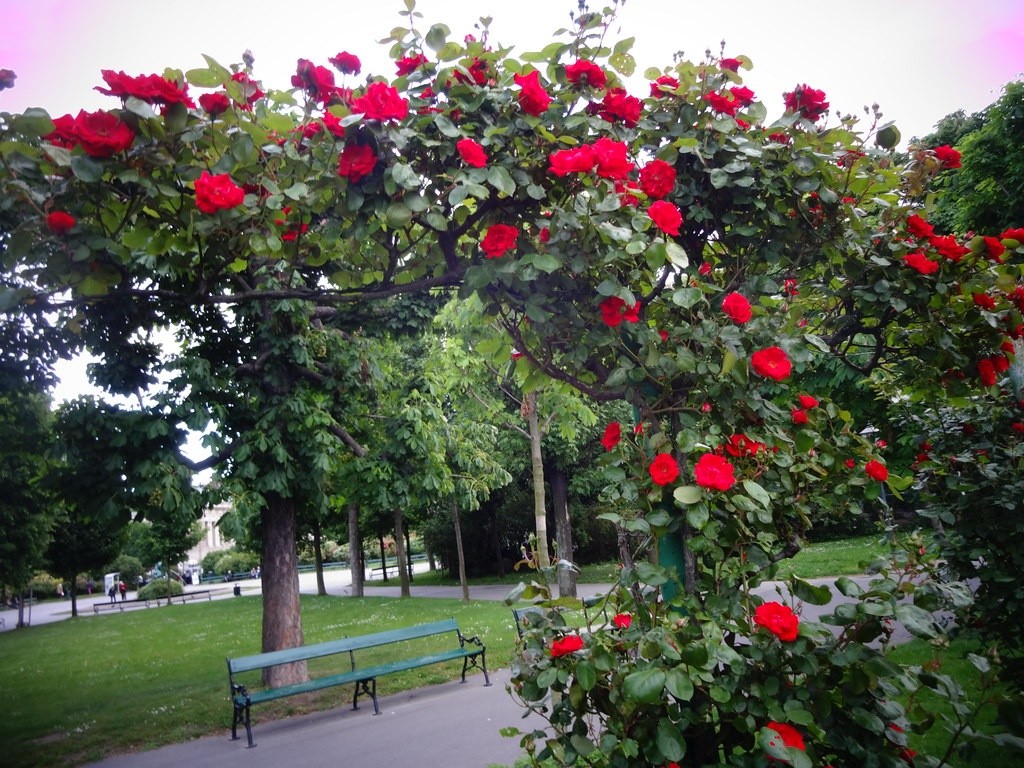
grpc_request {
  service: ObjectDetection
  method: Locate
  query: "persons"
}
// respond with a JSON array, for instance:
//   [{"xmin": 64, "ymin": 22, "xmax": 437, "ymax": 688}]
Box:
[
  {"xmin": 7, "ymin": 595, "xmax": 19, "ymax": 608},
  {"xmin": 250, "ymin": 566, "xmax": 262, "ymax": 578},
  {"xmin": 87, "ymin": 579, "xmax": 93, "ymax": 595},
  {"xmin": 57, "ymin": 583, "xmax": 64, "ymax": 599},
  {"xmin": 225, "ymin": 570, "xmax": 232, "ymax": 581},
  {"xmin": 137, "ymin": 569, "xmax": 190, "ymax": 588},
  {"xmin": 119, "ymin": 580, "xmax": 126, "ymax": 599},
  {"xmin": 109, "ymin": 584, "xmax": 115, "ymax": 603}
]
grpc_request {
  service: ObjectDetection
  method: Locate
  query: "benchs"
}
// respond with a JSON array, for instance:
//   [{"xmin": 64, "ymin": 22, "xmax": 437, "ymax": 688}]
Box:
[
  {"xmin": 226, "ymin": 616, "xmax": 493, "ymax": 749},
  {"xmin": 93, "ymin": 597, "xmax": 151, "ymax": 615},
  {"xmin": 201, "ymin": 572, "xmax": 251, "ymax": 584},
  {"xmin": 156, "ymin": 589, "xmax": 211, "ymax": 609},
  {"xmin": 24, "ymin": 598, "xmax": 38, "ymax": 606},
  {"xmin": 298, "ymin": 565, "xmax": 315, "ymax": 572},
  {"xmin": 323, "ymin": 561, "xmax": 346, "ymax": 570},
  {"xmin": 510, "ymin": 585, "xmax": 661, "ymax": 677},
  {"xmin": 366, "ymin": 554, "xmax": 427, "ymax": 567},
  {"xmin": 369, "ymin": 562, "xmax": 415, "ymax": 580}
]
